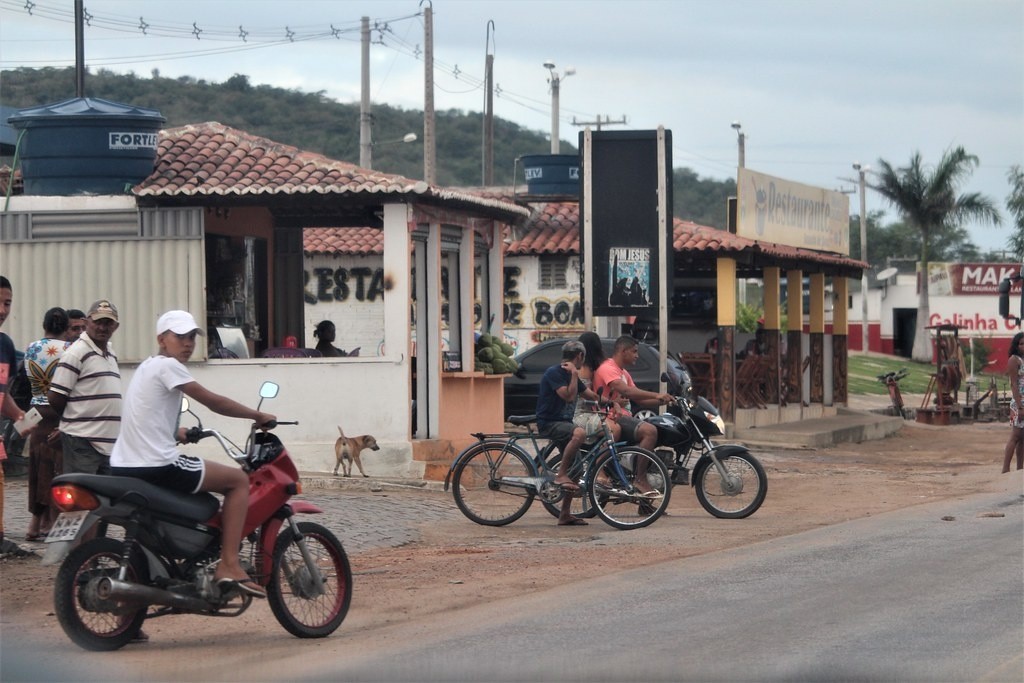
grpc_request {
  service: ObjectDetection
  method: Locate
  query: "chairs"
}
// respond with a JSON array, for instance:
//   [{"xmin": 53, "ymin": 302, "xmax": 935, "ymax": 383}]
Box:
[{"xmin": 678, "ymin": 351, "xmax": 810, "ymax": 410}]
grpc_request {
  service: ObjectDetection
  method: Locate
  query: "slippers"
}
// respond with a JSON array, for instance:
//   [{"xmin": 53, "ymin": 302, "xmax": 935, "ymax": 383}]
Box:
[
  {"xmin": 633, "ymin": 487, "xmax": 664, "ymax": 499},
  {"xmin": 131, "ymin": 629, "xmax": 149, "ymax": 642},
  {"xmin": 215, "ymin": 578, "xmax": 266, "ymax": 599},
  {"xmin": 557, "ymin": 516, "xmax": 589, "ymax": 525},
  {"xmin": 639, "ymin": 505, "xmax": 668, "ymax": 516},
  {"xmin": 552, "ymin": 481, "xmax": 580, "ymax": 491}
]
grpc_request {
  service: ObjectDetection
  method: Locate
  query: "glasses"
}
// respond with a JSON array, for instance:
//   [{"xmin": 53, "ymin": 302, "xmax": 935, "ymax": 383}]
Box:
[{"xmin": 69, "ymin": 325, "xmax": 86, "ymax": 332}]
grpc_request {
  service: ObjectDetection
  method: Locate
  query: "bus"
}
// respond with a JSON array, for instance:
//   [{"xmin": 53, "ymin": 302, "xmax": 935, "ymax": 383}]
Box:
[{"xmin": 998, "ymin": 256, "xmax": 1024, "ymax": 329}]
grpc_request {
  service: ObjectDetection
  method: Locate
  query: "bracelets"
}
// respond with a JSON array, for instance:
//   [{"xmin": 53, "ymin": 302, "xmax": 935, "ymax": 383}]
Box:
[{"xmin": 1018, "ymin": 407, "xmax": 1024, "ymax": 409}]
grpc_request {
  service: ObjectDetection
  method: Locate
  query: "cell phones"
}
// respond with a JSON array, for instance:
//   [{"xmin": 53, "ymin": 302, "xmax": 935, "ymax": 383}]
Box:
[{"xmin": 12, "ymin": 407, "xmax": 43, "ymax": 435}]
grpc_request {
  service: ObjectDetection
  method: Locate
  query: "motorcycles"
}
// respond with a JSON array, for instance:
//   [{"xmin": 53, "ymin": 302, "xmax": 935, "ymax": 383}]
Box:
[
  {"xmin": 531, "ymin": 373, "xmax": 769, "ymax": 519},
  {"xmin": 41, "ymin": 381, "xmax": 354, "ymax": 652}
]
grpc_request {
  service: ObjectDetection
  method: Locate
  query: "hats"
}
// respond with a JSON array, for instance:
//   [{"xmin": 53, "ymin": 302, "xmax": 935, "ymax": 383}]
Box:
[
  {"xmin": 87, "ymin": 299, "xmax": 120, "ymax": 323},
  {"xmin": 156, "ymin": 310, "xmax": 206, "ymax": 336}
]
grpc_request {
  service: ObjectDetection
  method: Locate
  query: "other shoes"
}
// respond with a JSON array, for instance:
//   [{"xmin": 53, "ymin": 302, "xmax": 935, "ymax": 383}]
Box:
[
  {"xmin": 596, "ymin": 477, "xmax": 614, "ymax": 488},
  {"xmin": 0, "ymin": 537, "xmax": 20, "ymax": 558}
]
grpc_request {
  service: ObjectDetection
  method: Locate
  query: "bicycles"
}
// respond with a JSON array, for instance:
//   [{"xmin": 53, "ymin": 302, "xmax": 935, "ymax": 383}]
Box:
[
  {"xmin": 443, "ymin": 386, "xmax": 672, "ymax": 530},
  {"xmin": 876, "ymin": 368, "xmax": 911, "ymax": 418}
]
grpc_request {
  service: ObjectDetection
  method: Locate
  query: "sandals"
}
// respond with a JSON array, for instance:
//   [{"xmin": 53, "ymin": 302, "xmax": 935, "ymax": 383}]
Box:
[{"xmin": 24, "ymin": 532, "xmax": 47, "ymax": 541}]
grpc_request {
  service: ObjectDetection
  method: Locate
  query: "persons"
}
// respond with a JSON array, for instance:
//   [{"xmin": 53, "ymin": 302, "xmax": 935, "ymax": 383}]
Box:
[
  {"xmin": 0, "ymin": 277, "xmax": 38, "ymax": 559},
  {"xmin": 593, "ymin": 337, "xmax": 677, "ymax": 515},
  {"xmin": 745, "ymin": 328, "xmax": 765, "ymax": 355},
  {"xmin": 47, "ymin": 299, "xmax": 122, "ymax": 474},
  {"xmin": 571, "ymin": 331, "xmax": 620, "ymax": 488},
  {"xmin": 314, "ymin": 320, "xmax": 343, "ymax": 357},
  {"xmin": 535, "ymin": 340, "xmax": 622, "ymax": 526},
  {"xmin": 1002, "ymin": 332, "xmax": 1024, "ymax": 474},
  {"xmin": 24, "ymin": 306, "xmax": 87, "ymax": 542},
  {"xmin": 108, "ymin": 309, "xmax": 277, "ymax": 599}
]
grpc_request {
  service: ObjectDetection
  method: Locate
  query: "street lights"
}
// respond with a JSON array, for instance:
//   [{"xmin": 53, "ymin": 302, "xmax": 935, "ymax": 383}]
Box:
[
  {"xmin": 850, "ymin": 161, "xmax": 869, "ymax": 350},
  {"xmin": 545, "ymin": 62, "xmax": 578, "ymax": 153},
  {"xmin": 731, "ymin": 121, "xmax": 746, "ymax": 164},
  {"xmin": 358, "ymin": 132, "xmax": 418, "ymax": 171}
]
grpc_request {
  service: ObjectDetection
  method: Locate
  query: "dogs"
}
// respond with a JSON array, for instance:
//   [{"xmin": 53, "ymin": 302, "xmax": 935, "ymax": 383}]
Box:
[{"xmin": 333, "ymin": 425, "xmax": 380, "ymax": 478}]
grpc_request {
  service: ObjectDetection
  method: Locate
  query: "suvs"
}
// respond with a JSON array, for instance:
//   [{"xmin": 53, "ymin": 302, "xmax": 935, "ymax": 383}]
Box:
[{"xmin": 503, "ymin": 337, "xmax": 691, "ymax": 421}]
